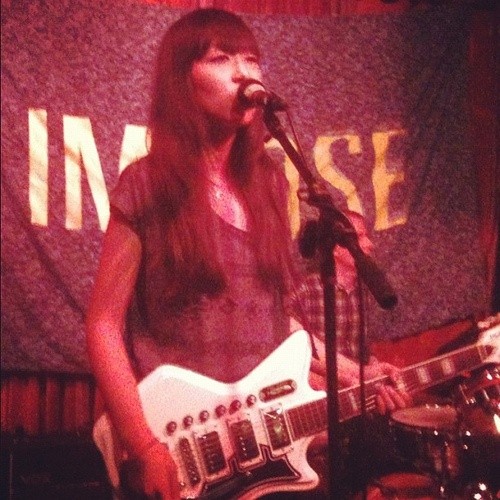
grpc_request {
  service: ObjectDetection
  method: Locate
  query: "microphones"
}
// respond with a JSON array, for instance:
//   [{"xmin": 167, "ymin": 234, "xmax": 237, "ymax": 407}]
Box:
[{"xmin": 237, "ymin": 79, "xmax": 288, "ymax": 112}]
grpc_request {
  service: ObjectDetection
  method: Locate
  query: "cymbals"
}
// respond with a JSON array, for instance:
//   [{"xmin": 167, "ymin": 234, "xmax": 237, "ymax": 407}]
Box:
[{"xmin": 457, "ymin": 368, "xmax": 500, "ymax": 437}]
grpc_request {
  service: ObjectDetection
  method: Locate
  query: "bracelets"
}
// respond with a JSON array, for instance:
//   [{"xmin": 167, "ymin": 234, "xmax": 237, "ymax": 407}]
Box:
[{"xmin": 131, "ymin": 436, "xmax": 159, "ymax": 460}]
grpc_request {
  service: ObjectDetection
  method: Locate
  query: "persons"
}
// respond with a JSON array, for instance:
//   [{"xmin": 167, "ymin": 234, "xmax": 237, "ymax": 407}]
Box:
[
  {"xmin": 292, "ymin": 209, "xmax": 385, "ymax": 490},
  {"xmin": 86, "ymin": 10, "xmax": 412, "ymax": 500}
]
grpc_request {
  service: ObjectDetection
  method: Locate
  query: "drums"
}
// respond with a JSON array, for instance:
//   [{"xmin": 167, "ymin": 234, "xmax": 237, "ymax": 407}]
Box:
[{"xmin": 389, "ymin": 405, "xmax": 477, "ymax": 482}]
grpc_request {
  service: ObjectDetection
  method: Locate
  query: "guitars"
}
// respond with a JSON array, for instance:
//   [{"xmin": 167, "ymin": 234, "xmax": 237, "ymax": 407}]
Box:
[{"xmin": 91, "ymin": 312, "xmax": 500, "ymax": 500}]
